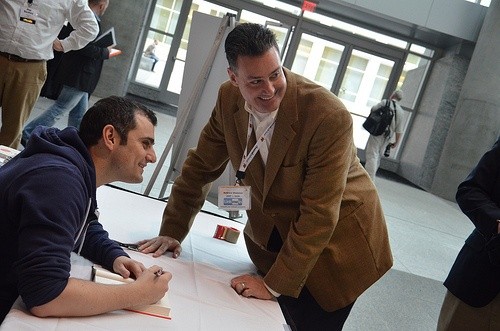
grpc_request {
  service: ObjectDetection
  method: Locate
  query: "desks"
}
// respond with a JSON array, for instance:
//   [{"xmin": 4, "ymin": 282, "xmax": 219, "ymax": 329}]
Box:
[{"xmin": 0, "ymin": 183, "xmax": 288, "ymax": 331}]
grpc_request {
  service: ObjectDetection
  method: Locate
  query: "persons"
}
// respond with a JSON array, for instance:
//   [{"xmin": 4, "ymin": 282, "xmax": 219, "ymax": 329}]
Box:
[
  {"xmin": 0, "ymin": 96, "xmax": 173, "ymax": 328},
  {"xmin": 366, "ymin": 89, "xmax": 404, "ymax": 180},
  {"xmin": 0, "ymin": 0, "xmax": 159, "ymax": 150},
  {"xmin": 435, "ymin": 136, "xmax": 500, "ymax": 331},
  {"xmin": 137, "ymin": 23, "xmax": 395, "ymax": 331}
]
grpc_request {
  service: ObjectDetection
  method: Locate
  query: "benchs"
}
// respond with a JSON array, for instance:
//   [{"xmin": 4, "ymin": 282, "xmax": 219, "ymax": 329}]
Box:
[{"xmin": 139, "ymin": 56, "xmax": 156, "ymax": 70}]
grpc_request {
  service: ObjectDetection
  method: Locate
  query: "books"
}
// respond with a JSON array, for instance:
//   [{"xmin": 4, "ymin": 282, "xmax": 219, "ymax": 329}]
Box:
[{"xmin": 92, "ymin": 265, "xmax": 171, "ymax": 319}]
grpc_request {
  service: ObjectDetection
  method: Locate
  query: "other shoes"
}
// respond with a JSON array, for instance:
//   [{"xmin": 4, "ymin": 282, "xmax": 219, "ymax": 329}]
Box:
[{"xmin": 20, "ymin": 138, "xmax": 26, "ymax": 147}]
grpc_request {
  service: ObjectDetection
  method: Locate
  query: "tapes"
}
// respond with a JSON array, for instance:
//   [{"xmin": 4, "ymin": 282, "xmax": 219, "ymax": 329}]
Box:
[{"xmin": 225, "ymin": 228, "xmax": 240, "ymax": 243}]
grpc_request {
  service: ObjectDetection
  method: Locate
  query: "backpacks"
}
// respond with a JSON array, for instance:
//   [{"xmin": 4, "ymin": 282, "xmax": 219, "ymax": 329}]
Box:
[{"xmin": 363, "ymin": 99, "xmax": 394, "ymax": 136}]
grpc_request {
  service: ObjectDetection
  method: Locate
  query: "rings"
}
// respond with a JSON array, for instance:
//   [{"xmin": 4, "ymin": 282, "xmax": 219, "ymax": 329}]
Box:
[{"xmin": 242, "ymin": 282, "xmax": 245, "ymax": 290}]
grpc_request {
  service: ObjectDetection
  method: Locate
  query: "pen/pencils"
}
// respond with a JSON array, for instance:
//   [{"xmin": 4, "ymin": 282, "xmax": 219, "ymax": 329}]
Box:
[{"xmin": 154, "ymin": 270, "xmax": 163, "ymax": 278}]
